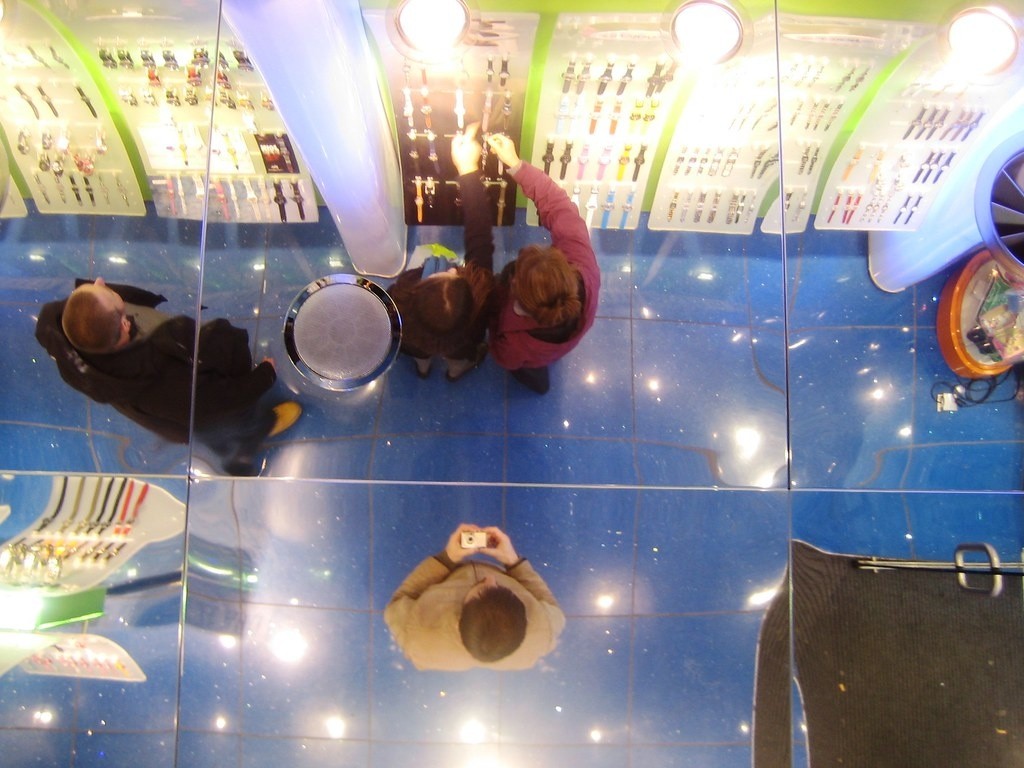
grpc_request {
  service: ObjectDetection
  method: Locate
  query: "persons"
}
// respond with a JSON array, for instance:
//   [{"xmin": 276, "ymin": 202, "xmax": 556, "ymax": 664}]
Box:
[
  {"xmin": 384, "ymin": 523, "xmax": 566, "ymax": 673},
  {"xmin": 35, "ymin": 276, "xmax": 303, "ymax": 472},
  {"xmin": 483, "ymin": 134, "xmax": 603, "ymax": 392},
  {"xmin": 387, "ymin": 125, "xmax": 495, "ymax": 382}
]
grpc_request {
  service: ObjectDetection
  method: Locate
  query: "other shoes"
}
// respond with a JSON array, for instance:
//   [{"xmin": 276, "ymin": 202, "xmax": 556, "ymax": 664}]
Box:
[
  {"xmin": 416, "ymin": 362, "xmax": 430, "ymax": 376},
  {"xmin": 447, "ymin": 341, "xmax": 488, "ymax": 381},
  {"xmin": 269, "ymin": 401, "xmax": 301, "ymax": 438}
]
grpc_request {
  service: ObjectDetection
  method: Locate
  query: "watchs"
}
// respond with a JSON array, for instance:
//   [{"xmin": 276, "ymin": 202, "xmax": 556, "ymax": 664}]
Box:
[
  {"xmin": 398, "ymin": 59, "xmax": 506, "ymax": 226},
  {"xmin": 16, "ymin": 48, "xmax": 131, "ymax": 209},
  {"xmin": 97, "ymin": 46, "xmax": 307, "ymax": 222},
  {"xmin": 827, "ymin": 107, "xmax": 987, "ymax": 225},
  {"xmin": 8, "ymin": 476, "xmax": 150, "ymax": 564},
  {"xmin": 541, "ymin": 52, "xmax": 675, "ymax": 232},
  {"xmin": 666, "ymin": 67, "xmax": 867, "ymax": 224}
]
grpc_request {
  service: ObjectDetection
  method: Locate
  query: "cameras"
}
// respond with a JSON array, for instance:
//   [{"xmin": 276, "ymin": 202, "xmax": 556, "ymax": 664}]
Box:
[{"xmin": 460, "ymin": 531, "xmax": 487, "ymax": 549}]
